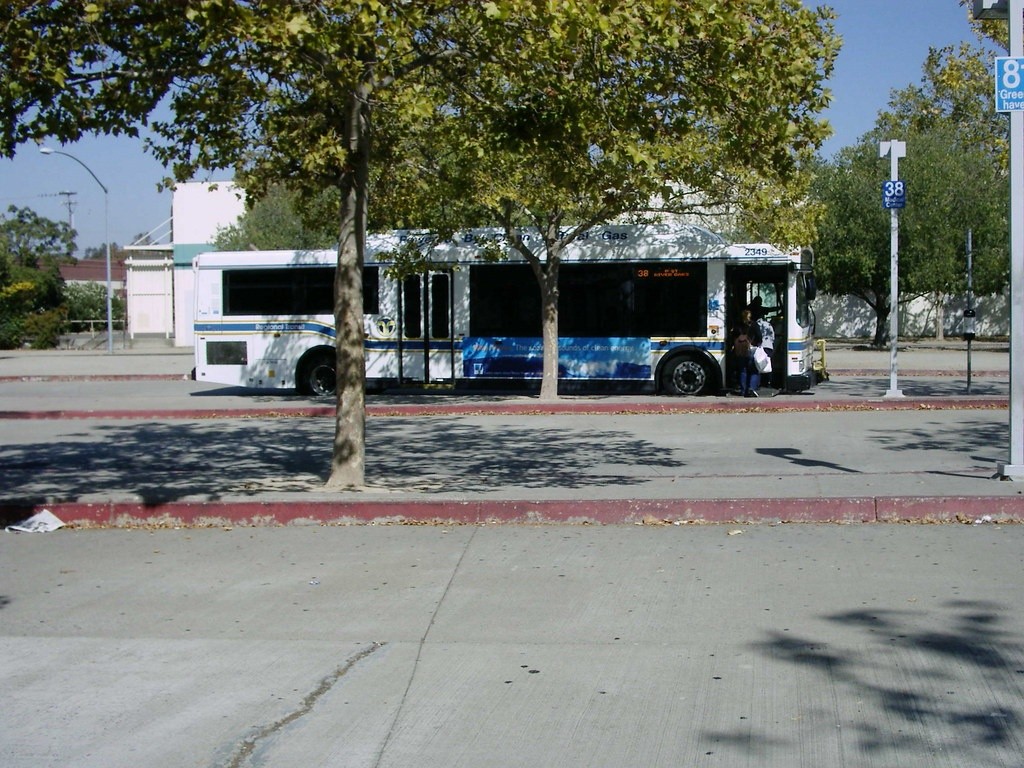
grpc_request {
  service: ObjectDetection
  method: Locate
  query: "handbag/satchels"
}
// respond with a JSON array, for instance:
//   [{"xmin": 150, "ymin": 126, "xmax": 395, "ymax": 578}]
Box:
[{"xmin": 753, "ymin": 345, "xmax": 772, "ymax": 373}]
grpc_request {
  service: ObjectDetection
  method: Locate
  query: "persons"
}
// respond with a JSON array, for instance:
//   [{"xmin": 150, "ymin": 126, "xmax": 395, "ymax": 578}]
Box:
[{"xmin": 729, "ymin": 296, "xmax": 782, "ymax": 397}]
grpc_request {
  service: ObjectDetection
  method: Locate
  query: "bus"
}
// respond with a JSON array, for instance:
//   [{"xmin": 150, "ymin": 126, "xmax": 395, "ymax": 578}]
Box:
[{"xmin": 183, "ymin": 225, "xmax": 830, "ymax": 398}]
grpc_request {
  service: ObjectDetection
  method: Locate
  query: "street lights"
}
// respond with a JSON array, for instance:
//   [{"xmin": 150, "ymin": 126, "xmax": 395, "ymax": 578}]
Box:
[{"xmin": 40, "ymin": 147, "xmax": 114, "ymax": 354}]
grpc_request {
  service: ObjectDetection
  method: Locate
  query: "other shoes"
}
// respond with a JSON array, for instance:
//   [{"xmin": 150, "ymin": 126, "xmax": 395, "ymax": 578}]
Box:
[
  {"xmin": 738, "ymin": 391, "xmax": 748, "ymax": 398},
  {"xmin": 747, "ymin": 390, "xmax": 759, "ymax": 397}
]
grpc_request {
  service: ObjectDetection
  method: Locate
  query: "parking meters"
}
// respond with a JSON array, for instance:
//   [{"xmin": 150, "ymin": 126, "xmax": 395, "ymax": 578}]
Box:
[{"xmin": 963, "ymin": 308, "xmax": 975, "ymax": 341}]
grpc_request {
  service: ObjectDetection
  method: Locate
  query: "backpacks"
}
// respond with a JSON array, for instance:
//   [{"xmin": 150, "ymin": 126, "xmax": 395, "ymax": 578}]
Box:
[{"xmin": 734, "ymin": 328, "xmax": 755, "ymax": 358}]
